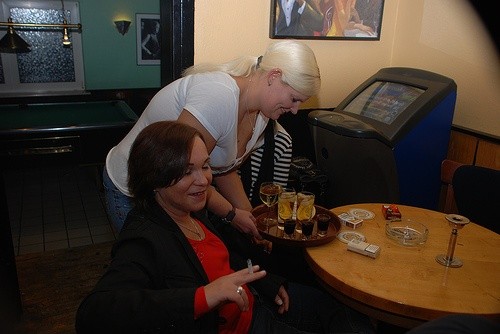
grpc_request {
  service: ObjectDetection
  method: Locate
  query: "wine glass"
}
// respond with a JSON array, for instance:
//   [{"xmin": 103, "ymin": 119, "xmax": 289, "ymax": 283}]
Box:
[{"xmin": 259, "ymin": 182, "xmax": 282, "ymax": 226}]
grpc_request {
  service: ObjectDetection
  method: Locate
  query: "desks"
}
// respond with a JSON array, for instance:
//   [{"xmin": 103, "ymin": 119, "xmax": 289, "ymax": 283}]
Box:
[
  {"xmin": 303, "ymin": 203, "xmax": 500, "ymax": 323},
  {"xmin": 0, "ymin": 99, "xmax": 138, "ymax": 167}
]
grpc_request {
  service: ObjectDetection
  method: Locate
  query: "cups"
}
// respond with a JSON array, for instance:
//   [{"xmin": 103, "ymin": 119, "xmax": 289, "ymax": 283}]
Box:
[
  {"xmin": 284, "ymin": 218, "xmax": 296, "ymax": 240},
  {"xmin": 296, "ymin": 192, "xmax": 315, "ymax": 234},
  {"xmin": 317, "ymin": 213, "xmax": 331, "ymax": 237},
  {"xmin": 278, "ymin": 188, "xmax": 296, "ymax": 230},
  {"xmin": 301, "ymin": 219, "xmax": 315, "ymax": 240}
]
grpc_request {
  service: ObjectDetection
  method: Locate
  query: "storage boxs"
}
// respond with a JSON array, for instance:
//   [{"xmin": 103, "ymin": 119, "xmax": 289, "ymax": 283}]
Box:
[
  {"xmin": 338, "ymin": 213, "xmax": 364, "ymax": 229},
  {"xmin": 348, "ymin": 239, "xmax": 380, "ymax": 259},
  {"xmin": 383, "ymin": 205, "xmax": 401, "ymax": 220}
]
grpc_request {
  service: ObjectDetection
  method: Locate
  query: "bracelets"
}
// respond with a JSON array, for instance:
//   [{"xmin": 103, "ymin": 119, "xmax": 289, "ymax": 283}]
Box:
[{"xmin": 220, "ymin": 205, "xmax": 236, "ymax": 225}]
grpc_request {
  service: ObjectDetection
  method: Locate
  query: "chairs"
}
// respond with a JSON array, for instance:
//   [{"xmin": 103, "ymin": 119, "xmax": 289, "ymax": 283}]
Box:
[{"xmin": 451, "ymin": 165, "xmax": 500, "ymax": 235}]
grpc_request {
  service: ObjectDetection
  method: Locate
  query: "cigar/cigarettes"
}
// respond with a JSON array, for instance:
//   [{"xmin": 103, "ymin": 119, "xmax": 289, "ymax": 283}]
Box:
[{"xmin": 246, "ymin": 258, "xmax": 254, "ymax": 274}]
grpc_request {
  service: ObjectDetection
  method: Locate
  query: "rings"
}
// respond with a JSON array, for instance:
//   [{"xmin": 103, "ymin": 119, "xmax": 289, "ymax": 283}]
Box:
[{"xmin": 236, "ymin": 285, "xmax": 243, "ymax": 295}]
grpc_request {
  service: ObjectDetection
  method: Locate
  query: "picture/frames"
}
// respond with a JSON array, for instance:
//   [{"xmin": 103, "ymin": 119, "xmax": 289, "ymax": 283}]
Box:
[
  {"xmin": 269, "ymin": 0, "xmax": 384, "ymax": 41},
  {"xmin": 135, "ymin": 13, "xmax": 160, "ymax": 66}
]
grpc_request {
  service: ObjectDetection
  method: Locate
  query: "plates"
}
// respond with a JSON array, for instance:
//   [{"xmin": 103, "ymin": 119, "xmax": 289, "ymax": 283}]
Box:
[{"xmin": 292, "ymin": 200, "xmax": 315, "ymax": 221}]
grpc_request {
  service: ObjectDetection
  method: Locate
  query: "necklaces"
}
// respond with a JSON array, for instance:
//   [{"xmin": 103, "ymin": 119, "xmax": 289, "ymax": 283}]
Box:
[
  {"xmin": 246, "ymin": 74, "xmax": 257, "ymax": 133},
  {"xmin": 177, "ymin": 218, "xmax": 202, "ymax": 239}
]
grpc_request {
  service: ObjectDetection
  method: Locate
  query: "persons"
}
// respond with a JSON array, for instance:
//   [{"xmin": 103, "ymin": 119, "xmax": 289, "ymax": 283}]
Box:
[
  {"xmin": 75, "ymin": 120, "xmax": 378, "ymax": 334},
  {"xmin": 102, "ymin": 41, "xmax": 322, "ymax": 254}
]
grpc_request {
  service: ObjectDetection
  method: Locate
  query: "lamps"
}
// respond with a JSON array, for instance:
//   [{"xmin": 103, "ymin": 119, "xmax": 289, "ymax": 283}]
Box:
[
  {"xmin": 0, "ymin": 18, "xmax": 32, "ymax": 52},
  {"xmin": 114, "ymin": 20, "xmax": 131, "ymax": 35}
]
